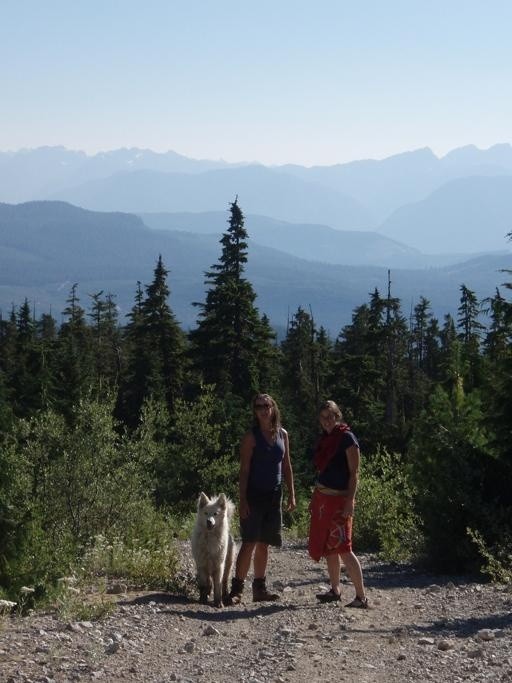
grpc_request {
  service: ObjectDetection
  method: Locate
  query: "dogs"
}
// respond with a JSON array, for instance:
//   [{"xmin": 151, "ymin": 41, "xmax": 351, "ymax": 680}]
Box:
[{"xmin": 189, "ymin": 491, "xmax": 237, "ymax": 607}]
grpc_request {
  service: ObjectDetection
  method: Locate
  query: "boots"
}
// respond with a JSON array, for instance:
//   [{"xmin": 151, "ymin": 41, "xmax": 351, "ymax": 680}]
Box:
[
  {"xmin": 252, "ymin": 577, "xmax": 280, "ymax": 602},
  {"xmin": 228, "ymin": 577, "xmax": 244, "ymax": 605}
]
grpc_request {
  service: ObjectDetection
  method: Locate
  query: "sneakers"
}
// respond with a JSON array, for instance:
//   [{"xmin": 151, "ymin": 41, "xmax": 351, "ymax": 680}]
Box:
[
  {"xmin": 316, "ymin": 589, "xmax": 342, "ymax": 603},
  {"xmin": 345, "ymin": 595, "xmax": 369, "ymax": 609}
]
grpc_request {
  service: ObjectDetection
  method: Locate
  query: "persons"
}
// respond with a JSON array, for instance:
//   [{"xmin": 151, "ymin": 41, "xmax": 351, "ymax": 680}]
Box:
[
  {"xmin": 307, "ymin": 401, "xmax": 370, "ymax": 609},
  {"xmin": 226, "ymin": 392, "xmax": 298, "ymax": 604}
]
